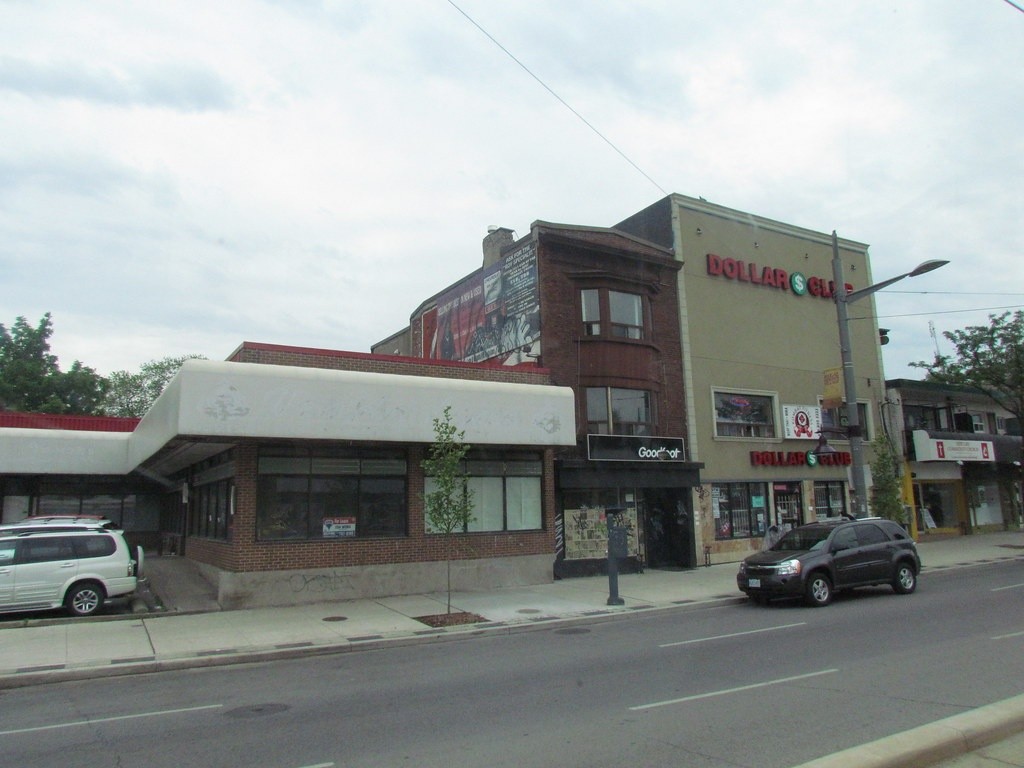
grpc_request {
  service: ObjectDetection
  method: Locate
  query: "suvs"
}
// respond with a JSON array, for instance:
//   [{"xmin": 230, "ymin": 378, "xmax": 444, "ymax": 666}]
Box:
[
  {"xmin": 0, "ymin": 516, "xmax": 144, "ymax": 617},
  {"xmin": 737, "ymin": 517, "xmax": 921, "ymax": 606}
]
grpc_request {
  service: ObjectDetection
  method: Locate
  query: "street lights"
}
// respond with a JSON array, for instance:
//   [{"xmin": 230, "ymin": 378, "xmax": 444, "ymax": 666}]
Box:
[{"xmin": 810, "ymin": 230, "xmax": 949, "ymax": 519}]
